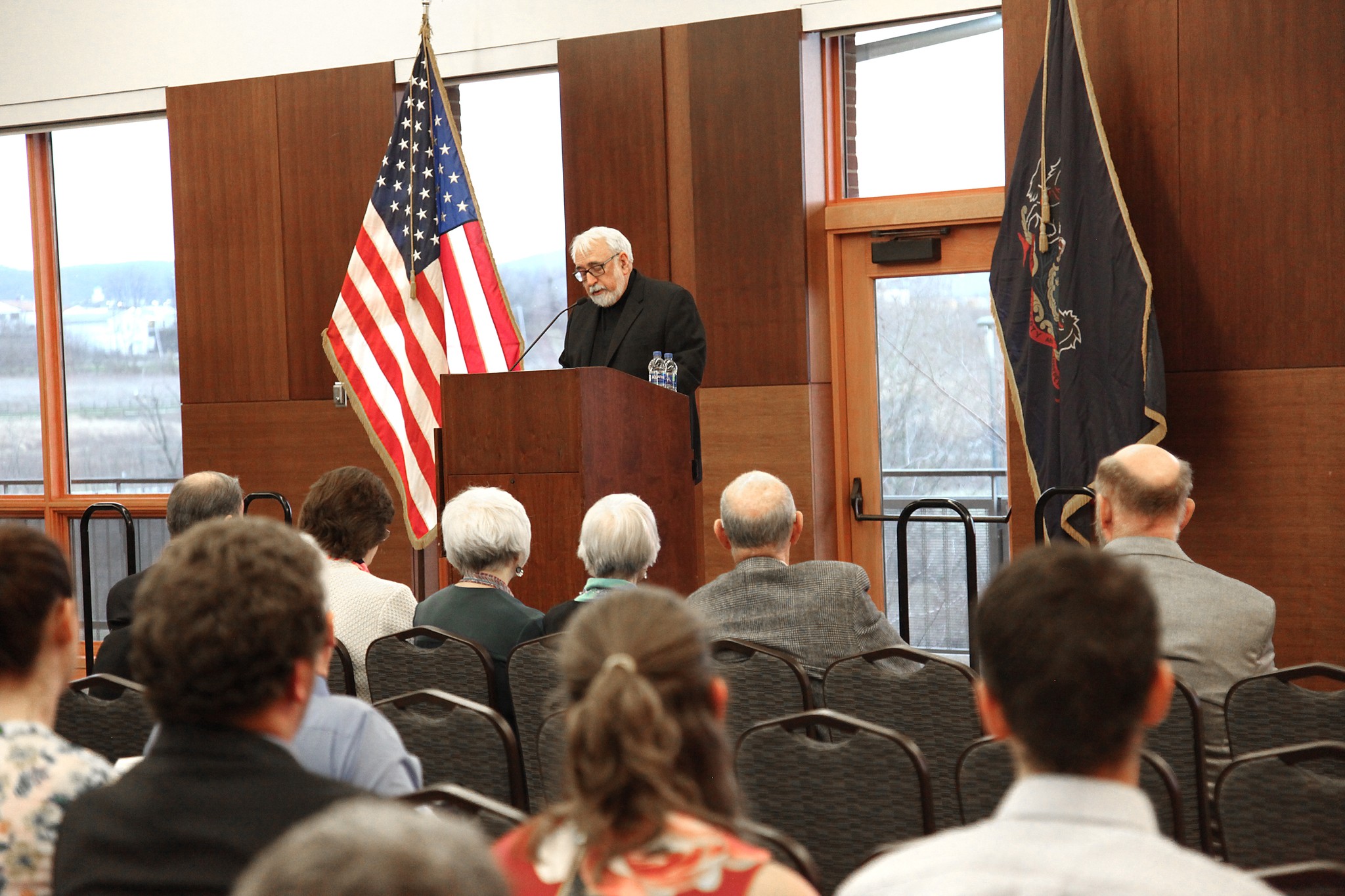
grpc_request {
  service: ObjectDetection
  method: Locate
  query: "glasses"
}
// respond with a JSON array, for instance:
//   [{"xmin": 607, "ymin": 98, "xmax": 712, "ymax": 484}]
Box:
[
  {"xmin": 572, "ymin": 253, "xmax": 620, "ymax": 282},
  {"xmin": 382, "ymin": 529, "xmax": 391, "ymax": 541}
]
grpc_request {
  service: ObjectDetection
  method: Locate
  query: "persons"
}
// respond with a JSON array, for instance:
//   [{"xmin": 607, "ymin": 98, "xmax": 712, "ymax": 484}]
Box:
[
  {"xmin": 686, "ymin": 470, "xmax": 923, "ymax": 738},
  {"xmin": 0, "ymin": 526, "xmax": 114, "ymax": 896},
  {"xmin": 88, "ymin": 471, "xmax": 244, "ymax": 702},
  {"xmin": 49, "ymin": 517, "xmax": 368, "ymax": 896},
  {"xmin": 493, "ymin": 587, "xmax": 822, "ymax": 896},
  {"xmin": 542, "ymin": 493, "xmax": 661, "ymax": 638},
  {"xmin": 558, "ymin": 226, "xmax": 706, "ymax": 486},
  {"xmin": 257, "ymin": 533, "xmax": 421, "ymax": 798},
  {"xmin": 1094, "ymin": 443, "xmax": 1279, "ymax": 856},
  {"xmin": 231, "ymin": 797, "xmax": 509, "ymax": 896},
  {"xmin": 294, "ymin": 466, "xmax": 419, "ymax": 705},
  {"xmin": 834, "ymin": 545, "xmax": 1284, "ymax": 896},
  {"xmin": 413, "ymin": 486, "xmax": 545, "ymax": 750}
]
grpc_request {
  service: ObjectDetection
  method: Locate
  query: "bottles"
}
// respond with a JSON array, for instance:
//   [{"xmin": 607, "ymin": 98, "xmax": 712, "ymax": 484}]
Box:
[
  {"xmin": 664, "ymin": 353, "xmax": 677, "ymax": 392},
  {"xmin": 648, "ymin": 351, "xmax": 667, "ymax": 388}
]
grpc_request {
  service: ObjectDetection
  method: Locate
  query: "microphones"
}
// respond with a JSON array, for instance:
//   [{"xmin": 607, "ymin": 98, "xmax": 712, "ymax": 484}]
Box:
[{"xmin": 508, "ymin": 295, "xmax": 588, "ymax": 371}]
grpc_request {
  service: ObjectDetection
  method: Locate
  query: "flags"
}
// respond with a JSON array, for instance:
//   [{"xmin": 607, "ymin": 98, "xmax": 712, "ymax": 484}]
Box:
[
  {"xmin": 323, "ymin": 25, "xmax": 526, "ymax": 550},
  {"xmin": 988, "ymin": 0, "xmax": 1169, "ymax": 549}
]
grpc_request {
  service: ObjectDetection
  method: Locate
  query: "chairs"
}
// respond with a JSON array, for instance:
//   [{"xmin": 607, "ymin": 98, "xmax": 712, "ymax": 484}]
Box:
[
  {"xmin": 370, "ymin": 688, "xmax": 530, "ymax": 840},
  {"xmin": 822, "ymin": 644, "xmax": 985, "ymax": 833},
  {"xmin": 956, "ymin": 734, "xmax": 1185, "ymax": 846},
  {"xmin": 736, "ymin": 712, "xmax": 933, "ymax": 896},
  {"xmin": 1224, "ymin": 660, "xmax": 1345, "ymax": 779},
  {"xmin": 507, "ymin": 629, "xmax": 576, "ymax": 815},
  {"xmin": 324, "ymin": 623, "xmax": 500, "ymax": 718},
  {"xmin": 53, "ymin": 673, "xmax": 157, "ymax": 763},
  {"xmin": 708, "ymin": 637, "xmax": 815, "ymax": 756},
  {"xmin": 1211, "ymin": 741, "xmax": 1345, "ymax": 896},
  {"xmin": 1141, "ymin": 677, "xmax": 1206, "ymax": 848}
]
